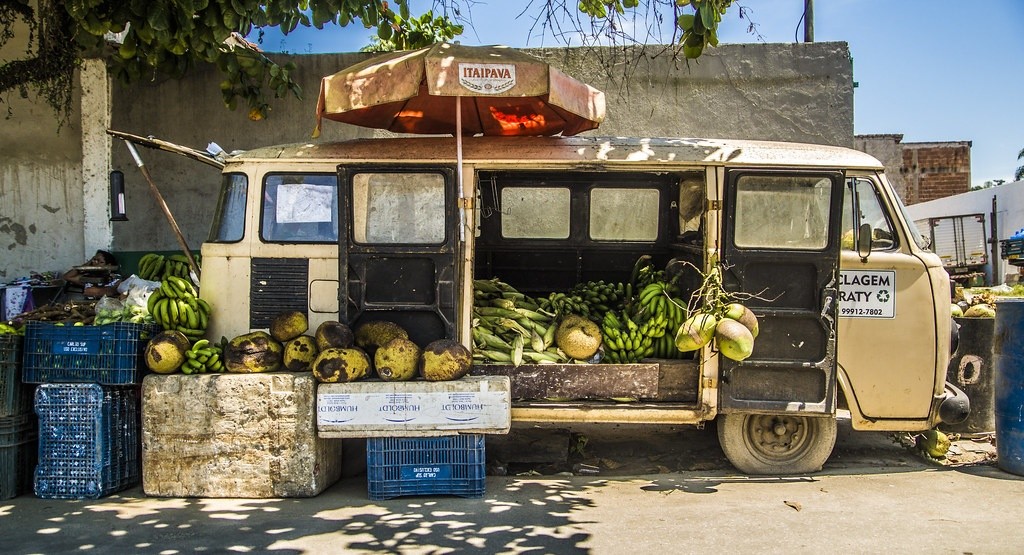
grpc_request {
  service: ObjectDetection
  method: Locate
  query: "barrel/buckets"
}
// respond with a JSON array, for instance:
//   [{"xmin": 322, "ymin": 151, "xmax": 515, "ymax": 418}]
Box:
[{"xmin": 992, "ymin": 298, "xmax": 1024, "ymax": 480}]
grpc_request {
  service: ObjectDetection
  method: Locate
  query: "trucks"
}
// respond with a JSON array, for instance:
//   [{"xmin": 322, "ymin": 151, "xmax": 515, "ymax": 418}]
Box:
[{"xmin": 913, "ymin": 212, "xmax": 989, "ymax": 299}]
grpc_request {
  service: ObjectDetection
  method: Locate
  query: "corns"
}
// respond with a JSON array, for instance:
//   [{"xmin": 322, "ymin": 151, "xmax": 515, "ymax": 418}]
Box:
[
  {"xmin": 34, "ymin": 306, "xmax": 152, "ymax": 376},
  {"xmin": 471, "ymin": 278, "xmax": 571, "ymax": 366},
  {"xmin": 0, "ymin": 323, "xmax": 25, "ymax": 336}
]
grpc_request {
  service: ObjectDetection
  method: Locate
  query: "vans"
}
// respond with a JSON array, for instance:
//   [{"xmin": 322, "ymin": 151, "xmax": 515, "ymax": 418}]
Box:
[{"xmin": 98, "ymin": 127, "xmax": 956, "ymax": 480}]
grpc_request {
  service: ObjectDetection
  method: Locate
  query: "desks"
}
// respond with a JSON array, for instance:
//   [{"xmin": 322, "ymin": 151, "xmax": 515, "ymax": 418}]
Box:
[{"xmin": 0, "ymin": 284, "xmax": 68, "ymax": 308}]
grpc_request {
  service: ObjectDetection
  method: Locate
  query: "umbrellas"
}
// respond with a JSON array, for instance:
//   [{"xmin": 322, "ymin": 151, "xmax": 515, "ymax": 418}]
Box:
[{"xmin": 316, "ymin": 40, "xmax": 607, "ymax": 242}]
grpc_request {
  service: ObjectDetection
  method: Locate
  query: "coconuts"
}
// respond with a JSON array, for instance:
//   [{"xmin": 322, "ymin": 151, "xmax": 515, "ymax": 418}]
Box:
[{"xmin": 675, "ymin": 303, "xmax": 759, "ymax": 362}]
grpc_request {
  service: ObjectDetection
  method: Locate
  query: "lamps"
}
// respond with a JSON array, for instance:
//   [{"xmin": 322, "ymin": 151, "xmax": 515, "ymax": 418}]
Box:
[{"xmin": 109, "ymin": 170, "xmax": 128, "ymax": 222}]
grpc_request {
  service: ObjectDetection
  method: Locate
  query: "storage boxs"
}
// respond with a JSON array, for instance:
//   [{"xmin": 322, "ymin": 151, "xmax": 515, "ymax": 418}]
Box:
[
  {"xmin": 141, "ymin": 371, "xmax": 342, "ymax": 499},
  {"xmin": 366, "ymin": 434, "xmax": 486, "ymax": 501},
  {"xmin": 21, "ymin": 320, "xmax": 162, "ymax": 386},
  {"xmin": 0, "ymin": 334, "xmax": 38, "ymax": 501},
  {"xmin": 317, "ymin": 375, "xmax": 511, "ymax": 438},
  {"xmin": 34, "ymin": 384, "xmax": 141, "ymax": 500}
]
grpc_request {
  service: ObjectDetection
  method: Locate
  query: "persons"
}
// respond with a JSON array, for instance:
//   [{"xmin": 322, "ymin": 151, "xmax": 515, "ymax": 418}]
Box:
[{"xmin": 63, "ymin": 249, "xmax": 122, "ymax": 297}]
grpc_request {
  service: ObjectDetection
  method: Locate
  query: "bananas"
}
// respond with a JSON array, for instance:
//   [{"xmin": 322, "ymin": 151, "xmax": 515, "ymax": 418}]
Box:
[
  {"xmin": 539, "ymin": 266, "xmax": 691, "ymax": 364},
  {"xmin": 138, "ymin": 254, "xmax": 225, "ymax": 375}
]
